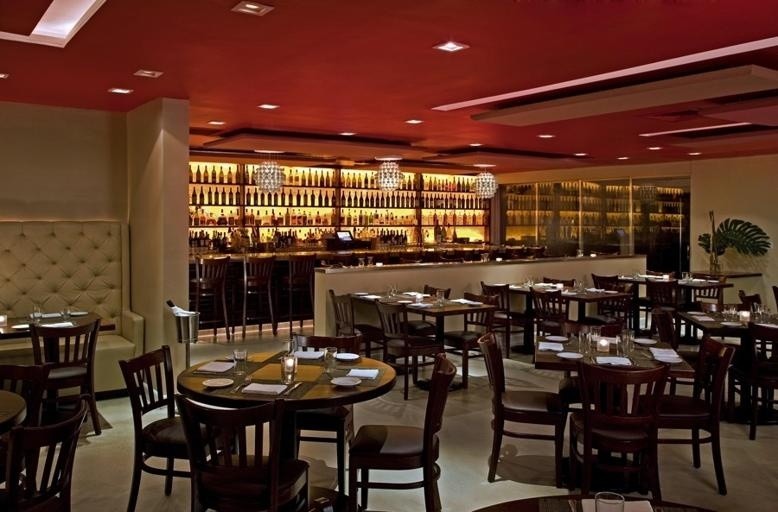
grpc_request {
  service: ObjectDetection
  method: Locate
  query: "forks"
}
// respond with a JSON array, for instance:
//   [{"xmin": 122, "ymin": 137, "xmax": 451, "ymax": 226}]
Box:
[{"xmin": 231, "ymin": 375, "xmax": 253, "ymax": 395}]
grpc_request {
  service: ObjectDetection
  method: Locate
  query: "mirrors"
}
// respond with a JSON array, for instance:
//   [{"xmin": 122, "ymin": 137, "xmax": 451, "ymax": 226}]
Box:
[{"xmin": 500, "ymin": 175, "xmax": 691, "ymax": 274}]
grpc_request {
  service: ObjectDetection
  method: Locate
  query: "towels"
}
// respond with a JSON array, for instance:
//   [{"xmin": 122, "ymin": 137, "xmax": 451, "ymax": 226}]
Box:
[
  {"xmin": 242, "ymin": 381, "xmax": 289, "ymax": 398},
  {"xmin": 196, "ymin": 361, "xmax": 236, "ymax": 372}
]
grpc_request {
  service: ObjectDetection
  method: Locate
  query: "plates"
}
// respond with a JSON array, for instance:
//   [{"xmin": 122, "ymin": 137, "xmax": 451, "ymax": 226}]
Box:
[
  {"xmin": 493, "ymin": 284, "xmax": 618, "ymax": 297},
  {"xmin": 545, "ymin": 336, "xmax": 683, "ymax": 364},
  {"xmin": 354, "ymin": 291, "xmax": 484, "ymax": 309},
  {"xmin": 11, "ymin": 311, "xmax": 88, "ymax": 330},
  {"xmin": 201, "ymin": 352, "xmax": 361, "ymax": 388},
  {"xmin": 687, "ymin": 311, "xmax": 778, "ymax": 329},
  {"xmin": 618, "ymin": 274, "xmax": 720, "ymax": 284}
]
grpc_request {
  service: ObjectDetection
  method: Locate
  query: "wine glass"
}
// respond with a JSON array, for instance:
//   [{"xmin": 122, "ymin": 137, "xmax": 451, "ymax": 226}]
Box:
[{"xmin": 574, "ymin": 281, "xmax": 584, "ymax": 298}]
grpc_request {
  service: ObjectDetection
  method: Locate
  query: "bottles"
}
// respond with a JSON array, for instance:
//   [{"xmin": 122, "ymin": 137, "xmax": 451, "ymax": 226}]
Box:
[
  {"xmin": 721, "ymin": 303, "xmax": 771, "ymax": 323},
  {"xmin": 167, "ymin": 300, "xmax": 187, "ymax": 316},
  {"xmin": 374, "ymin": 242, "xmax": 428, "ymax": 263},
  {"xmin": 191, "ymin": 186, "xmax": 483, "ymax": 208},
  {"xmin": 189, "ymin": 164, "xmax": 476, "ymax": 192},
  {"xmin": 189, "ymin": 226, "xmax": 458, "ymax": 248},
  {"xmin": 188, "ymin": 206, "xmax": 484, "ymax": 226},
  {"xmin": 504, "ymin": 183, "xmax": 685, "ymax": 243}
]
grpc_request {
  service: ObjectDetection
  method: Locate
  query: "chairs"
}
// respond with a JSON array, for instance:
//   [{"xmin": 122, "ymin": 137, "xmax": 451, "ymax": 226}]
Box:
[
  {"xmin": 232, "ymin": 254, "xmax": 278, "ymax": 338},
  {"xmin": 189, "ymin": 256, "xmax": 231, "ymax": 340},
  {"xmin": 1, "ymin": 364, "xmax": 50, "ymax": 426},
  {"xmin": 29, "ymin": 318, "xmax": 102, "ymax": 435},
  {"xmin": 278, "ymin": 253, "xmax": 317, "ymax": 337},
  {"xmin": 173, "ymin": 393, "xmax": 310, "ymax": 512},
  {"xmin": 320, "ymin": 245, "xmax": 545, "ymax": 264},
  {"xmin": 118, "ymin": 345, "xmax": 237, "ymax": 511},
  {"xmin": 1, "ymin": 393, "xmax": 91, "ymax": 512}
]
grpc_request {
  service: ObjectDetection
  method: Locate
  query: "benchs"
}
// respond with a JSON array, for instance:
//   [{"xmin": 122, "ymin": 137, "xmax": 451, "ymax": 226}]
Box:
[{"xmin": 0, "ymin": 220, "xmax": 144, "ymax": 399}]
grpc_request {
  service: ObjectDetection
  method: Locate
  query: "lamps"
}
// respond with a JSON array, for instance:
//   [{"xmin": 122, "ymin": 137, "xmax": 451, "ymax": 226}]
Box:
[
  {"xmin": 374, "ymin": 156, "xmax": 405, "ymax": 194},
  {"xmin": 471, "ymin": 167, "xmax": 498, "ymax": 200},
  {"xmin": 255, "ymin": 148, "xmax": 284, "ymax": 194}
]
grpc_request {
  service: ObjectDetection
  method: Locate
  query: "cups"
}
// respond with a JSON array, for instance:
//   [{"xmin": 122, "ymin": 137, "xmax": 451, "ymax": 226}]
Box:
[
  {"xmin": 521, "ymin": 275, "xmax": 536, "ymax": 290},
  {"xmin": 232, "ymin": 338, "xmax": 337, "ymax": 385},
  {"xmin": 416, "ymin": 287, "xmax": 425, "ymax": 303},
  {"xmin": 357, "ymin": 257, "xmax": 374, "ymax": 269},
  {"xmin": 388, "ymin": 282, "xmax": 399, "ymax": 298},
  {"xmin": 578, "ymin": 326, "xmax": 635, "ymax": 358},
  {"xmin": 33, "ymin": 304, "xmax": 71, "ymax": 321},
  {"xmin": 437, "ymin": 290, "xmax": 445, "ymax": 307},
  {"xmin": 596, "ymin": 492, "xmax": 626, "ymax": 512}
]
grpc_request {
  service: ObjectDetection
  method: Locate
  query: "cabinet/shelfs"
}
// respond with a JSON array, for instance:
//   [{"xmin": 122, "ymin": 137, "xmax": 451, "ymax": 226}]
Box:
[
  {"xmin": 340, "ymin": 166, "xmax": 421, "ymax": 245},
  {"xmin": 244, "ymin": 163, "xmax": 340, "ymax": 254},
  {"xmin": 421, "ymin": 172, "xmax": 490, "ymax": 245},
  {"xmin": 501, "ymin": 181, "xmax": 686, "ymax": 242},
  {"xmin": 187, "ymin": 152, "xmax": 244, "ymax": 254}
]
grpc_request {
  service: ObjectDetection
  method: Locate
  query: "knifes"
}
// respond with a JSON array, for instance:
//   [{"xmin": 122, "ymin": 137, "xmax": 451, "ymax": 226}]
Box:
[{"xmin": 193, "ymin": 371, "xmax": 229, "ymax": 377}]
